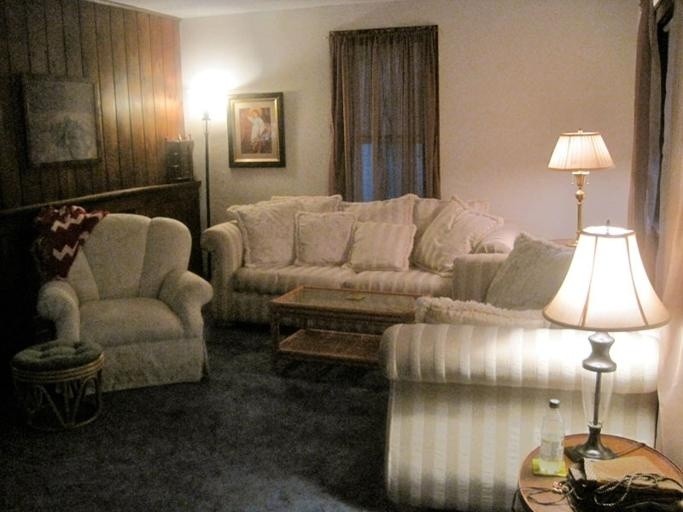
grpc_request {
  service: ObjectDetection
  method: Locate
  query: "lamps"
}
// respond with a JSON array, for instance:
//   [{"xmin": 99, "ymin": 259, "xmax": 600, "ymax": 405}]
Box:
[
  {"xmin": 548, "ymin": 130, "xmax": 615, "ymax": 247},
  {"xmin": 542, "ymin": 226, "xmax": 671, "ymax": 463}
]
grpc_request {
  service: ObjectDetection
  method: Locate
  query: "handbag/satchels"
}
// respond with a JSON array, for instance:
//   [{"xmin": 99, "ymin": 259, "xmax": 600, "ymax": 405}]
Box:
[{"xmin": 559, "ymin": 457, "xmax": 683, "ymax": 511}]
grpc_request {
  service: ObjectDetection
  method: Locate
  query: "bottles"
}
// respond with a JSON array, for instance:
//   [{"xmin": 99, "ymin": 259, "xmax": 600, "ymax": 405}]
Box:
[{"xmin": 538, "ymin": 399, "xmax": 565, "ymax": 475}]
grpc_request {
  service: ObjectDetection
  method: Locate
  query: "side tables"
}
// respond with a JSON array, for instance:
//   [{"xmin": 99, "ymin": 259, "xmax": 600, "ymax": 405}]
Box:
[{"xmin": 519, "ymin": 434, "xmax": 683, "ymax": 512}]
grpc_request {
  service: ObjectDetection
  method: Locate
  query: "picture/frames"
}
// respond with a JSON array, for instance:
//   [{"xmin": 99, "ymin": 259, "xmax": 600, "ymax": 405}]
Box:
[
  {"xmin": 227, "ymin": 93, "xmax": 285, "ymax": 167},
  {"xmin": 19, "ymin": 74, "xmax": 102, "ymax": 171}
]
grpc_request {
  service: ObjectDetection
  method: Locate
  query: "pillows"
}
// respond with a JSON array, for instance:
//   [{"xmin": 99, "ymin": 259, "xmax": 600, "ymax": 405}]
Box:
[
  {"xmin": 484, "ymin": 233, "xmax": 574, "ymax": 329},
  {"xmin": 226, "ymin": 195, "xmax": 505, "ymax": 278},
  {"xmin": 414, "ymin": 295, "xmax": 552, "ymax": 328}
]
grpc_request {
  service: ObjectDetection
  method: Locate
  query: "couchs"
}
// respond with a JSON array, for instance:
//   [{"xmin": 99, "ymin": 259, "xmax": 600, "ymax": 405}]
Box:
[
  {"xmin": 200, "ymin": 220, "xmax": 514, "ymax": 328},
  {"xmin": 379, "ymin": 253, "xmax": 659, "ymax": 512}
]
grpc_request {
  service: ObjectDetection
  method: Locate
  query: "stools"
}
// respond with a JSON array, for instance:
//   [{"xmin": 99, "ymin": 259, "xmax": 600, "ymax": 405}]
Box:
[{"xmin": 10, "ymin": 337, "xmax": 106, "ymax": 433}]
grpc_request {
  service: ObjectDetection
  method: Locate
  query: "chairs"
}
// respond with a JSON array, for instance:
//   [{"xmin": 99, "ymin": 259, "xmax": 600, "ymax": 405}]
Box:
[{"xmin": 38, "ymin": 212, "xmax": 214, "ymax": 399}]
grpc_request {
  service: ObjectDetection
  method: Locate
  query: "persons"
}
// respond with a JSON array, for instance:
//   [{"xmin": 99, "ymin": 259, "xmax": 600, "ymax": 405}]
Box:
[{"xmin": 246, "ymin": 109, "xmax": 266, "ymax": 152}]
